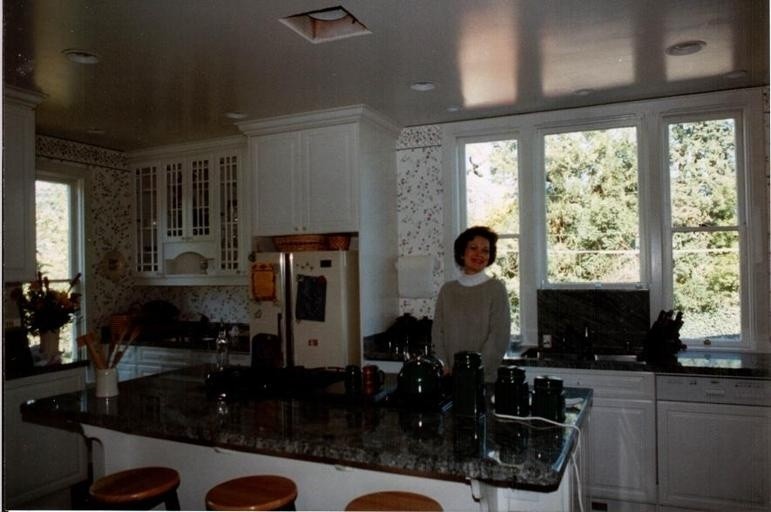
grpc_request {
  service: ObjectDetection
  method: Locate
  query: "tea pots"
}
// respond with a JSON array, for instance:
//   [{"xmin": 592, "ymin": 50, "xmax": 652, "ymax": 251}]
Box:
[{"xmin": 397, "ymin": 350, "xmax": 445, "ymax": 405}]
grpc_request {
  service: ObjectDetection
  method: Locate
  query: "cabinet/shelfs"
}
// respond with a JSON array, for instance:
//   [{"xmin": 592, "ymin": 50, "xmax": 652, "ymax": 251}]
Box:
[
  {"xmin": 88, "ymin": 345, "xmax": 136, "ymax": 384},
  {"xmin": 247, "ymin": 123, "xmax": 398, "ymax": 236},
  {"xmin": 4, "ymin": 374, "xmax": 79, "ymax": 499},
  {"xmin": 519, "ymin": 366, "xmax": 656, "ymax": 504},
  {"xmin": 164, "ymin": 150, "xmax": 212, "ymax": 244},
  {"xmin": 128, "ymin": 147, "xmax": 165, "ymax": 278},
  {"xmin": 655, "ymin": 399, "xmax": 771, "ymax": 512},
  {"xmin": 136, "ymin": 347, "xmax": 192, "ymax": 378},
  {"xmin": 212, "ymin": 146, "xmax": 249, "ymax": 276},
  {"xmin": 2, "ymin": 96, "xmax": 38, "ymax": 283}
]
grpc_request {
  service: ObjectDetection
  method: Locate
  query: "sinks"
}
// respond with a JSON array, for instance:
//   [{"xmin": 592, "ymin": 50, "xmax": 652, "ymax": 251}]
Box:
[{"xmin": 519, "ymin": 347, "xmax": 657, "ymax": 371}]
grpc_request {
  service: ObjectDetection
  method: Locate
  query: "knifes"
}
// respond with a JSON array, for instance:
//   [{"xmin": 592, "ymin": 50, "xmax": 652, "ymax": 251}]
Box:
[{"xmin": 648, "ymin": 309, "xmax": 684, "ymax": 348}]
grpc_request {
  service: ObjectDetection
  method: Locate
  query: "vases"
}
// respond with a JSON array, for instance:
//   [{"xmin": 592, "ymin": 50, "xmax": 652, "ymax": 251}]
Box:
[{"xmin": 39, "ymin": 324, "xmax": 60, "ymax": 354}]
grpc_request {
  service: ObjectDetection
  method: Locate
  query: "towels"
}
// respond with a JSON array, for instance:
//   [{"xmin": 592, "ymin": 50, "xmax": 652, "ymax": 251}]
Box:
[{"xmin": 398, "ymin": 252, "xmax": 435, "ymax": 299}]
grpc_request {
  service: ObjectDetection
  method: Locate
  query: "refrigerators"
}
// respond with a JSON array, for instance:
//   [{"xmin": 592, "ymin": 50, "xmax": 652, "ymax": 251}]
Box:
[{"xmin": 248, "ymin": 250, "xmax": 361, "ymax": 368}]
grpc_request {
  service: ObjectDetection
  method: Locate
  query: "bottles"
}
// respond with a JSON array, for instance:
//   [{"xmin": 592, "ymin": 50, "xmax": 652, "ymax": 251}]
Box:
[
  {"xmin": 495, "ymin": 365, "xmax": 530, "ymax": 417},
  {"xmin": 531, "ymin": 376, "xmax": 566, "ymax": 427},
  {"xmin": 450, "ymin": 350, "xmax": 487, "ymax": 415}
]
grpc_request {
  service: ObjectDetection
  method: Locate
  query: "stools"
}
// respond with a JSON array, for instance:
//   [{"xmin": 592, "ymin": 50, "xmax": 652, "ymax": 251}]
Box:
[
  {"xmin": 345, "ymin": 491, "xmax": 443, "ymax": 512},
  {"xmin": 89, "ymin": 466, "xmax": 182, "ymax": 510},
  {"xmin": 204, "ymin": 474, "xmax": 298, "ymax": 511}
]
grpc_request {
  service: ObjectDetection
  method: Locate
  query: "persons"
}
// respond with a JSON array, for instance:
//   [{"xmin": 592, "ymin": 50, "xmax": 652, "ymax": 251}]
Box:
[{"xmin": 431, "ymin": 228, "xmax": 510, "ymax": 385}]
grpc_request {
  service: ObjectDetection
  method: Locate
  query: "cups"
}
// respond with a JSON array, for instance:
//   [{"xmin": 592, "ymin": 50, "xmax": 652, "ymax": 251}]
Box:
[
  {"xmin": 94, "ymin": 366, "xmax": 120, "ymax": 399},
  {"xmin": 361, "ymin": 363, "xmax": 386, "ymax": 395},
  {"xmin": 343, "ymin": 364, "xmax": 362, "ymax": 393}
]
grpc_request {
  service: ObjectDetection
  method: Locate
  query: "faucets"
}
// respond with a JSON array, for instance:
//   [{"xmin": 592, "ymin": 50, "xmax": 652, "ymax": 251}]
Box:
[{"xmin": 579, "ymin": 326, "xmax": 596, "ymax": 361}]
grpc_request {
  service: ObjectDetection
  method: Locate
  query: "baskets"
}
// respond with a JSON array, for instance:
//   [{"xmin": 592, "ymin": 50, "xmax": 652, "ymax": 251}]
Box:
[{"xmin": 111, "ymin": 302, "xmax": 143, "ymax": 344}]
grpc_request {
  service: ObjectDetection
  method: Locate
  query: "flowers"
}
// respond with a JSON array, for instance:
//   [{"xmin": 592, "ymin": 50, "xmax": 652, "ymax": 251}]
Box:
[{"xmin": 11, "ymin": 270, "xmax": 82, "ymax": 336}]
